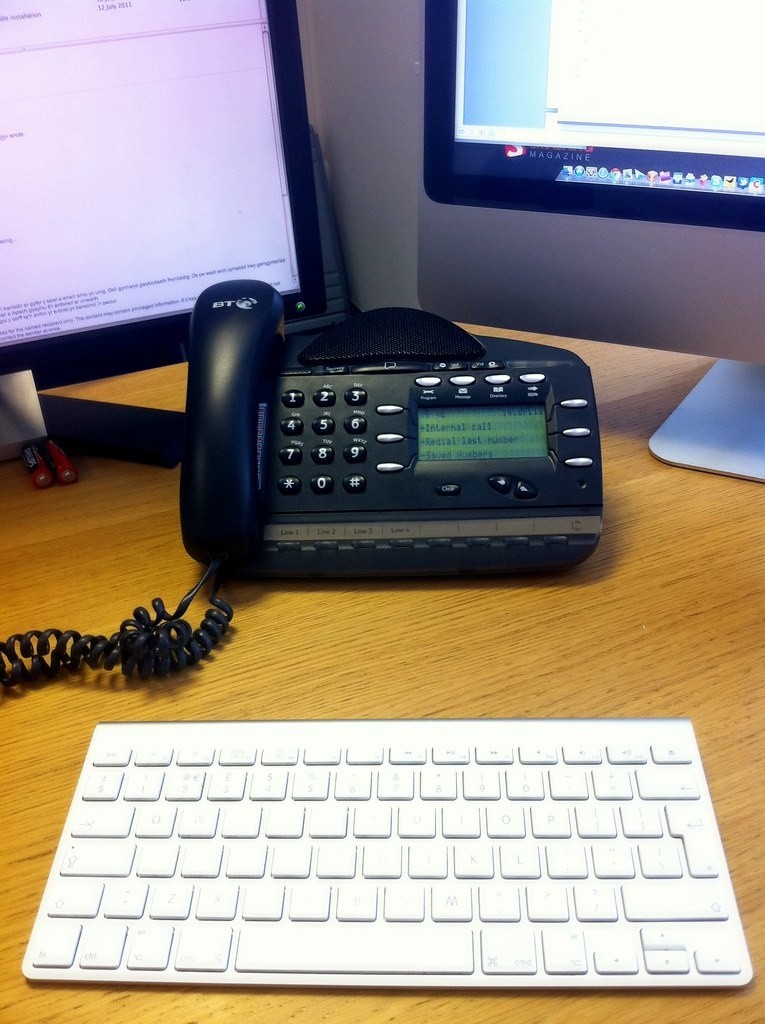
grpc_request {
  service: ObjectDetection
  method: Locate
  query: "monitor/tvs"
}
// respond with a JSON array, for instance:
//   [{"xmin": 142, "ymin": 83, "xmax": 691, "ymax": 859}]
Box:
[
  {"xmin": 0, "ymin": 0, "xmax": 325, "ymax": 375},
  {"xmin": 422, "ymin": 0, "xmax": 765, "ymax": 236}
]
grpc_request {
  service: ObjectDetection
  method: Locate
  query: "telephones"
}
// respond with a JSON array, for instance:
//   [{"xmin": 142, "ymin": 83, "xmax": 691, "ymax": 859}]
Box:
[{"xmin": 179, "ymin": 278, "xmax": 604, "ymax": 584}]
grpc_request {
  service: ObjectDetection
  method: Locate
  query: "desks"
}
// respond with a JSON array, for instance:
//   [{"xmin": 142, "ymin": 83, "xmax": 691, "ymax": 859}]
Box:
[{"xmin": 0, "ymin": 321, "xmax": 765, "ymax": 1024}]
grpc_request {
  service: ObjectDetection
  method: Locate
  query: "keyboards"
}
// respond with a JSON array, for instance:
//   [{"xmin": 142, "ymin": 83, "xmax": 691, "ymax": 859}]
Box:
[{"xmin": 21, "ymin": 716, "xmax": 754, "ymax": 990}]
